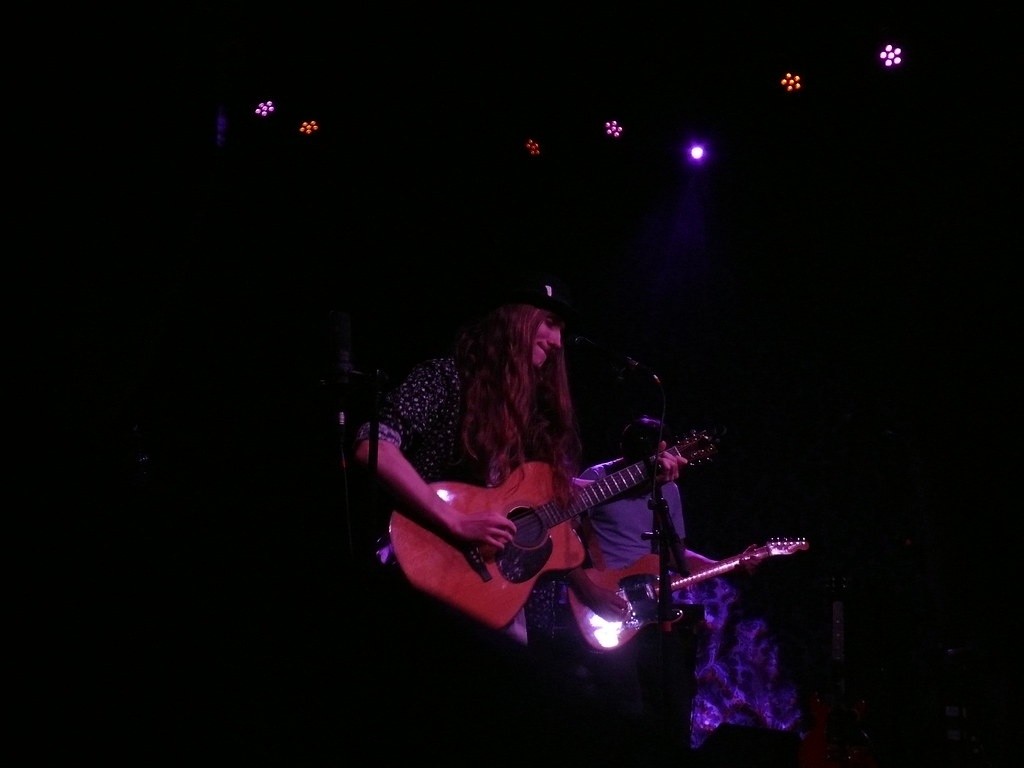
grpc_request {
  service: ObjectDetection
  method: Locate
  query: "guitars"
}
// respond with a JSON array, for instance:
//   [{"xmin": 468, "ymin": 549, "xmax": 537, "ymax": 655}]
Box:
[
  {"xmin": 384, "ymin": 426, "xmax": 722, "ymax": 640},
  {"xmin": 560, "ymin": 533, "xmax": 810, "ymax": 657}
]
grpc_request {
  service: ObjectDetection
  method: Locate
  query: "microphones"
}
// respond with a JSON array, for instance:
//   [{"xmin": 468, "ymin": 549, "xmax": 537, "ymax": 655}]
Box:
[
  {"xmin": 334, "ymin": 308, "xmax": 351, "ymax": 442},
  {"xmin": 575, "ymin": 337, "xmax": 662, "ymax": 385}
]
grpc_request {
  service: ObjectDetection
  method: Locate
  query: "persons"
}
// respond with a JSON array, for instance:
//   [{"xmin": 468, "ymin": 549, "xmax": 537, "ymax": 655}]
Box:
[
  {"xmin": 578, "ymin": 418, "xmax": 764, "ymax": 727},
  {"xmin": 347, "ymin": 283, "xmax": 688, "ymax": 718}
]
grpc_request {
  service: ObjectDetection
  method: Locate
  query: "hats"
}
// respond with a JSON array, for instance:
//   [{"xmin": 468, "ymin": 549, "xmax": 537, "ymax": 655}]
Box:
[{"xmin": 494, "ymin": 274, "xmax": 576, "ymax": 322}]
